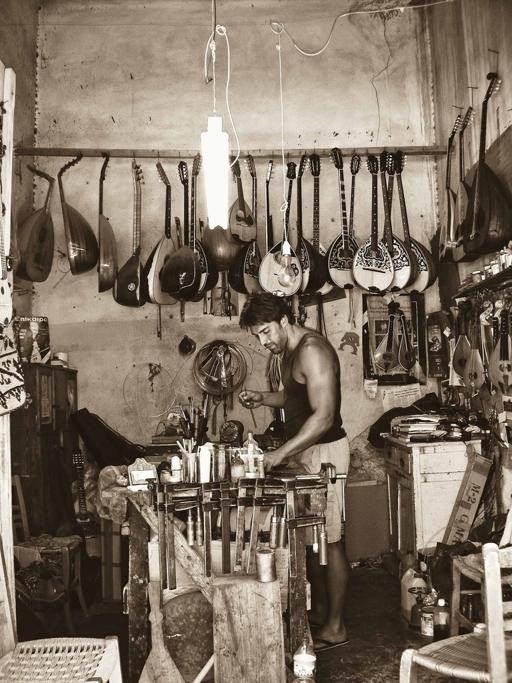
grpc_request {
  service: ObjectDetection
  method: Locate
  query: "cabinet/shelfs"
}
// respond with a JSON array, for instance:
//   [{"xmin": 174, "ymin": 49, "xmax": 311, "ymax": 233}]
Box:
[
  {"xmin": 11, "ymin": 361, "xmax": 102, "ymax": 633},
  {"xmin": 380, "ymin": 432, "xmax": 485, "ymax": 560}
]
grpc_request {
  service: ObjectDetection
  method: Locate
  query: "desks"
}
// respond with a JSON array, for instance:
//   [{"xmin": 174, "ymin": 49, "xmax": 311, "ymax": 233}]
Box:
[{"xmin": 95, "ymin": 441, "xmax": 328, "ymax": 683}]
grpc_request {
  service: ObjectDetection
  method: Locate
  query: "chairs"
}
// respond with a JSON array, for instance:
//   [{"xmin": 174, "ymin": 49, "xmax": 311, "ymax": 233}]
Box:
[
  {"xmin": 449, "ymin": 508, "xmax": 512, "ymax": 638},
  {"xmin": 12, "ymin": 474, "xmax": 89, "ymax": 634},
  {"xmin": 0, "ymin": 544, "xmax": 123, "ymax": 683},
  {"xmin": 399, "ymin": 542, "xmax": 512, "ymax": 683}
]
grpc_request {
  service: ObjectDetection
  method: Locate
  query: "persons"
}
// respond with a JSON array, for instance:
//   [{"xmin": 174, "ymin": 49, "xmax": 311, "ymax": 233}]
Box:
[{"xmin": 237, "ymin": 290, "xmax": 350, "ymax": 650}]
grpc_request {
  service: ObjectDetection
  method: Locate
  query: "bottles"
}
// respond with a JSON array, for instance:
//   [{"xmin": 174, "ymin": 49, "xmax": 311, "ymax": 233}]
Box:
[
  {"xmin": 230, "ymin": 453, "xmax": 246, "ymax": 484},
  {"xmin": 292, "ymin": 637, "xmax": 317, "ymax": 683},
  {"xmin": 242, "ymin": 432, "xmax": 259, "ymax": 479},
  {"xmin": 198, "ymin": 444, "xmax": 229, "ymax": 483},
  {"xmin": 434, "ymin": 597, "xmax": 449, "ymax": 641}
]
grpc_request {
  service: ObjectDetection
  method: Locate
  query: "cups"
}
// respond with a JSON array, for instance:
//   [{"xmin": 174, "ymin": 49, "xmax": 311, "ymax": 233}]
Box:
[{"xmin": 182, "ymin": 453, "xmax": 197, "ymax": 484}]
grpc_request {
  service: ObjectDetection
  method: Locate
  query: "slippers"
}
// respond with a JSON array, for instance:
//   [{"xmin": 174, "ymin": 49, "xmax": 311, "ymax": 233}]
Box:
[
  {"xmin": 305, "ymin": 610, "xmax": 322, "ymax": 629},
  {"xmin": 309, "ymin": 634, "xmax": 349, "ymax": 653}
]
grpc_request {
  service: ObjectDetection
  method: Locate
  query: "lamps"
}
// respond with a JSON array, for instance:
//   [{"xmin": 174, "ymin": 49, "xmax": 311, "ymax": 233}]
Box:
[
  {"xmin": 201, "ymin": 0, "xmax": 230, "ymax": 230},
  {"xmin": 277, "ymin": 11, "xmax": 297, "ymax": 286}
]
